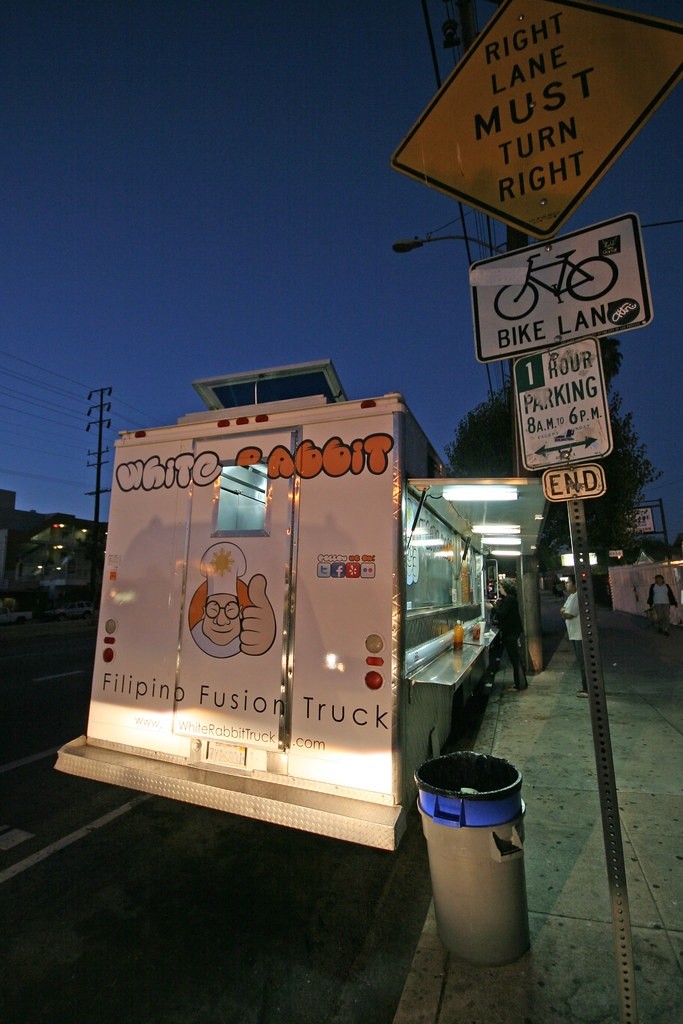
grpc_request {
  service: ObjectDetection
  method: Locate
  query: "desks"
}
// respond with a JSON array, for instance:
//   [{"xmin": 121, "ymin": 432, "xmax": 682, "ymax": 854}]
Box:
[{"xmin": 405, "ymin": 620, "xmax": 501, "ymax": 706}]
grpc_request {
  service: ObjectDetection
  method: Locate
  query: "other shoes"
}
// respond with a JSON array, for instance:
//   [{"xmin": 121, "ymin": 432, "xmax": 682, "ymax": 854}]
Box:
[
  {"xmin": 663, "ymin": 632, "xmax": 671, "ymax": 637},
  {"xmin": 657, "ymin": 626, "xmax": 662, "ymax": 634},
  {"xmin": 576, "ymin": 691, "xmax": 588, "ymax": 698},
  {"xmin": 508, "ymin": 683, "xmax": 518, "ymax": 691}
]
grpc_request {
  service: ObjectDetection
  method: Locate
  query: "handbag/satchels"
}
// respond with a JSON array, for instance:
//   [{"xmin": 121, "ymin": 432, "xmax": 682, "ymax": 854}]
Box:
[{"xmin": 645, "ymin": 606, "xmax": 657, "ymax": 623}]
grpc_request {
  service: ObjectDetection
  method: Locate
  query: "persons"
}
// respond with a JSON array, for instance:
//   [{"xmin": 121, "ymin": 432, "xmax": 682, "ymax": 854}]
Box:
[
  {"xmin": 557, "ymin": 575, "xmax": 588, "ymax": 696},
  {"xmin": 485, "ymin": 578, "xmax": 529, "ymax": 691},
  {"xmin": 647, "ymin": 574, "xmax": 678, "ymax": 637},
  {"xmin": 552, "ymin": 581, "xmax": 564, "ymax": 604}
]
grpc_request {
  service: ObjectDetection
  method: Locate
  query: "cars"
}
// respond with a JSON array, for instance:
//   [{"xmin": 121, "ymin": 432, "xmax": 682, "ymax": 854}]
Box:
[{"xmin": 51, "ymin": 601, "xmax": 95, "ymax": 621}]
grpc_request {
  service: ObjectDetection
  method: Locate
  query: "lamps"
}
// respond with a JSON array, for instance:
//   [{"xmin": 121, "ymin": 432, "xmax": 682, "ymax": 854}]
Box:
[{"xmin": 442, "ymin": 486, "xmax": 522, "ymax": 558}]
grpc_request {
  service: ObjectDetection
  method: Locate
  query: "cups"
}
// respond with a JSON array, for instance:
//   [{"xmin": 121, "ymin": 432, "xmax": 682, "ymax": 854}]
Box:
[
  {"xmin": 473, "ymin": 624, "xmax": 481, "ymax": 641},
  {"xmin": 479, "ymin": 622, "xmax": 486, "ymax": 633}
]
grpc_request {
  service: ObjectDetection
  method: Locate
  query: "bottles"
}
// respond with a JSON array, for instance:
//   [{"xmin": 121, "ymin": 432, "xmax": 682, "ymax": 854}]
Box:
[{"xmin": 454, "ymin": 620, "xmax": 464, "ymax": 650}]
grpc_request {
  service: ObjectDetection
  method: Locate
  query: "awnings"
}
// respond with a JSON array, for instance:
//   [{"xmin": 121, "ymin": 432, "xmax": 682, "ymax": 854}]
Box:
[{"xmin": 405, "ymin": 476, "xmax": 550, "ymax": 581}]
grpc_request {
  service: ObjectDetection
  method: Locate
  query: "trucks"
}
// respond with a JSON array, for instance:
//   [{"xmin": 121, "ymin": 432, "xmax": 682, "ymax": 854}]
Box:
[
  {"xmin": 53, "ymin": 358, "xmax": 551, "ymax": 853},
  {"xmin": 0, "ymin": 607, "xmax": 33, "ymax": 625}
]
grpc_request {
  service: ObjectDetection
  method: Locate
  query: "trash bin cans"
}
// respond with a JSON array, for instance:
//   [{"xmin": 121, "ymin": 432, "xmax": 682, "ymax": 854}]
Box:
[{"xmin": 412, "ymin": 751, "xmax": 531, "ymax": 968}]
